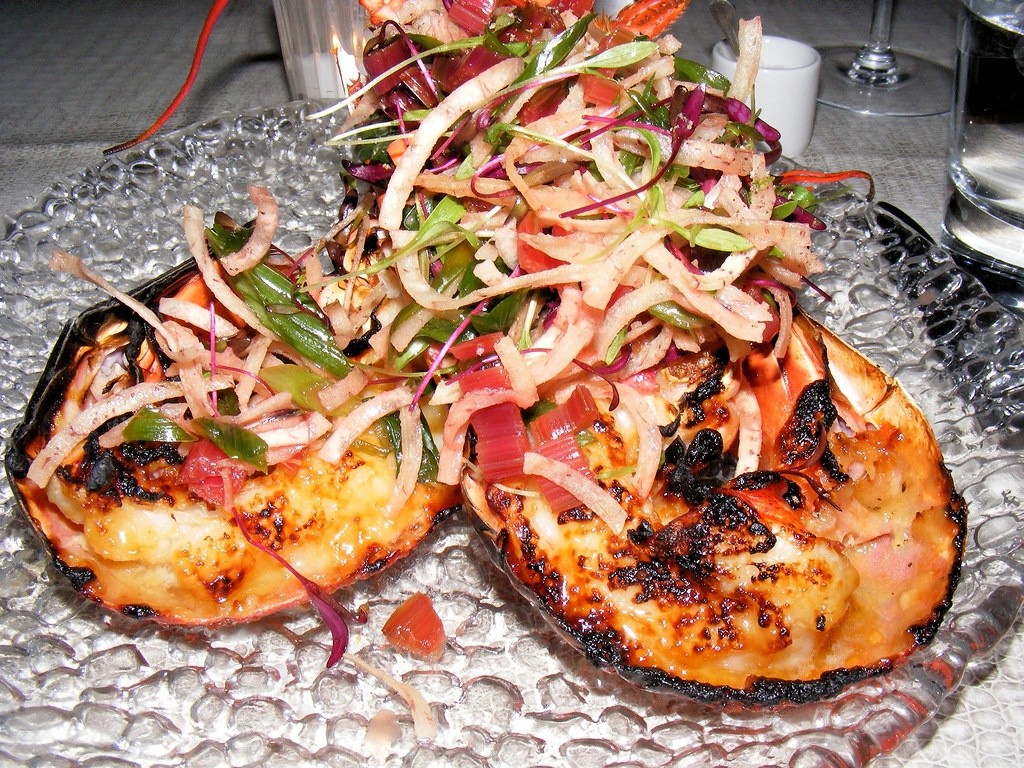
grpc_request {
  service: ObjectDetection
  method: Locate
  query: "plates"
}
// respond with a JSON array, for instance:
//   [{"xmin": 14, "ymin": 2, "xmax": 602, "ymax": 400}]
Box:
[{"xmin": 0, "ymin": 95, "xmax": 1024, "ymax": 768}]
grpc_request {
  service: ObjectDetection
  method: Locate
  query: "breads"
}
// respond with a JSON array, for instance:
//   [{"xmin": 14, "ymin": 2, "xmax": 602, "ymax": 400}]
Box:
[
  {"xmin": 461, "ymin": 323, "xmax": 965, "ymax": 706},
  {"xmin": 4, "ymin": 249, "xmax": 473, "ymax": 629}
]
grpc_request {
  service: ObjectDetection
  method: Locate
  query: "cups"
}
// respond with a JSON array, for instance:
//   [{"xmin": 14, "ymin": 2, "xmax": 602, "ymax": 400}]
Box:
[
  {"xmin": 274, "ymin": 0, "xmax": 399, "ymax": 102},
  {"xmin": 704, "ymin": 34, "xmax": 821, "ymax": 161},
  {"xmin": 940, "ymin": 0, "xmax": 1024, "ymax": 291}
]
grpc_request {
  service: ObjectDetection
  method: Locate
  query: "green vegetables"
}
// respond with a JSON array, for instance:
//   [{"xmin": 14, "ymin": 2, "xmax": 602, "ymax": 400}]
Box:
[{"xmin": 114, "ymin": 12, "xmax": 812, "ymax": 485}]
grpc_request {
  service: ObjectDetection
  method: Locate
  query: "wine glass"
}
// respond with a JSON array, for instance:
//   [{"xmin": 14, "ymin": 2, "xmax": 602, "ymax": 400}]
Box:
[{"xmin": 805, "ymin": 0, "xmax": 956, "ymax": 119}]
